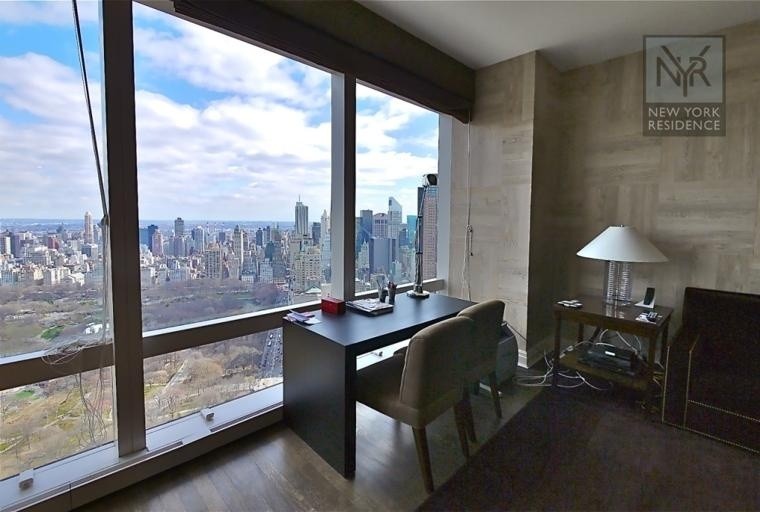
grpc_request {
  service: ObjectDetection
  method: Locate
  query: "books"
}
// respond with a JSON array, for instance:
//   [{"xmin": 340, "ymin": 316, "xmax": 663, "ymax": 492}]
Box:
[{"xmin": 345, "ymin": 298, "xmax": 394, "ymax": 318}]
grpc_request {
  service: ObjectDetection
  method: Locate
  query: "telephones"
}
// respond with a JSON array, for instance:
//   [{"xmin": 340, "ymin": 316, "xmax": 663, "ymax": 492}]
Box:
[{"xmin": 632, "ymin": 286, "xmax": 657, "ymax": 309}]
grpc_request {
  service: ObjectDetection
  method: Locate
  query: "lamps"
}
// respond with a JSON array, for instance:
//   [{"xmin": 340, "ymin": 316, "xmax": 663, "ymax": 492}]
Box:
[{"xmin": 575, "ymin": 227, "xmax": 668, "ymax": 307}]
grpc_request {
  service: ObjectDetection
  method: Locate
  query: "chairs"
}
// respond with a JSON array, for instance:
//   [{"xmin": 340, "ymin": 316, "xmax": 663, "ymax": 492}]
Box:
[
  {"xmin": 394, "ymin": 299, "xmax": 505, "ymax": 442},
  {"xmin": 355, "ymin": 316, "xmax": 478, "ymax": 493}
]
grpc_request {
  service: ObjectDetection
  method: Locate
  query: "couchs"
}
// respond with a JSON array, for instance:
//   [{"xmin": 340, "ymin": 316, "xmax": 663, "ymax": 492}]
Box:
[{"xmin": 662, "ymin": 287, "xmax": 760, "ymax": 454}]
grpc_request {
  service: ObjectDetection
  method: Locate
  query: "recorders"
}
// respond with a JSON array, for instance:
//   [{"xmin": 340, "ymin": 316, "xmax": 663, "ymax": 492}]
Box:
[{"xmin": 588, "ymin": 344, "xmax": 636, "ymax": 371}]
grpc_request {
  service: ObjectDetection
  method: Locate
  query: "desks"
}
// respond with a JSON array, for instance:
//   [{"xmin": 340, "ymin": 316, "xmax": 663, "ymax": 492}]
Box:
[
  {"xmin": 282, "ymin": 291, "xmax": 479, "ymax": 479},
  {"xmin": 551, "ymin": 293, "xmax": 674, "ymax": 412}
]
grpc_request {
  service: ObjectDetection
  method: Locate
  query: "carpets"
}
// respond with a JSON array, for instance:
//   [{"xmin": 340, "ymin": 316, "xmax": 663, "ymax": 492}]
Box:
[{"xmin": 413, "ymin": 364, "xmax": 759, "ymax": 512}]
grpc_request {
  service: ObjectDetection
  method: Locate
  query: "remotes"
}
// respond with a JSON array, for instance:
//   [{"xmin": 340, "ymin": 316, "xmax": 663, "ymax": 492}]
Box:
[{"xmin": 646, "ymin": 311, "xmax": 659, "ymax": 322}]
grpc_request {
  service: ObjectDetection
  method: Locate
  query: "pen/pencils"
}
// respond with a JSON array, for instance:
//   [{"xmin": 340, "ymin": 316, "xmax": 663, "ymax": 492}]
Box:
[
  {"xmin": 389, "ymin": 278, "xmax": 399, "ymax": 304},
  {"xmin": 375, "ymin": 277, "xmax": 387, "ymax": 301}
]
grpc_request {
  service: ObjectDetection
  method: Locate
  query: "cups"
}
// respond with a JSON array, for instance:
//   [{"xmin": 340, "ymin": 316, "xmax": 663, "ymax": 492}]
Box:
[
  {"xmin": 378, "ymin": 289, "xmax": 388, "ymax": 303},
  {"xmin": 388, "ymin": 289, "xmax": 397, "ymax": 305}
]
